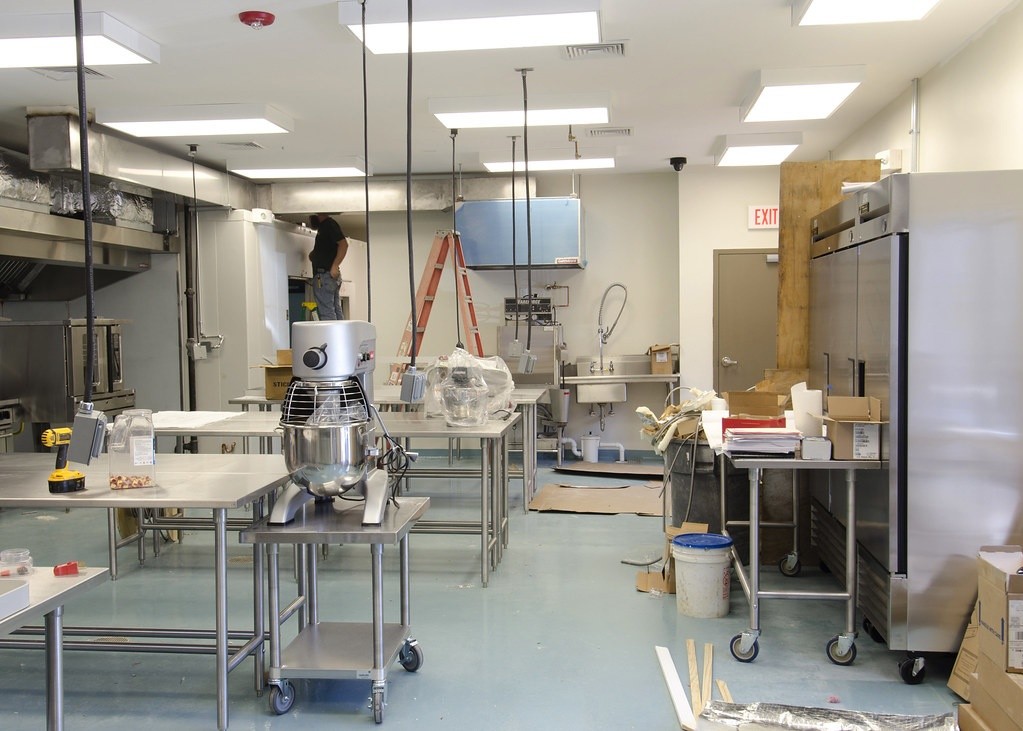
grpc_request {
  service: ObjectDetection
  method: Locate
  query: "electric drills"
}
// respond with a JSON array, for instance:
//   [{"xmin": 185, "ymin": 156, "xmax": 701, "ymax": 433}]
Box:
[{"xmin": 41, "ymin": 427, "xmax": 86, "ymax": 493}]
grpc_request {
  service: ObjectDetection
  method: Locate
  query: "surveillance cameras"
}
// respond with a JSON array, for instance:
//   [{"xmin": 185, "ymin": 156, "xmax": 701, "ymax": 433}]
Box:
[{"xmin": 670, "ymin": 157, "xmax": 687, "ymax": 171}]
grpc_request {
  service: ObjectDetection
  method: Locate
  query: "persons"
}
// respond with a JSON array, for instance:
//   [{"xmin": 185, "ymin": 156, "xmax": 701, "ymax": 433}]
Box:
[{"xmin": 308, "ymin": 214, "xmax": 349, "ymax": 321}]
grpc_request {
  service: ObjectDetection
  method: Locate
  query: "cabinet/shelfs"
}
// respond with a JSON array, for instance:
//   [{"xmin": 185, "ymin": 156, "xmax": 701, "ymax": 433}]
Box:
[{"xmin": 809, "ymin": 168, "xmax": 1023, "ymax": 688}]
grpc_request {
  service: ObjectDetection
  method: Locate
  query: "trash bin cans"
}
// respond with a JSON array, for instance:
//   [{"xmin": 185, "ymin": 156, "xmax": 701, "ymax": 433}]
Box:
[{"xmin": 668, "ymin": 438, "xmax": 751, "ymax": 565}]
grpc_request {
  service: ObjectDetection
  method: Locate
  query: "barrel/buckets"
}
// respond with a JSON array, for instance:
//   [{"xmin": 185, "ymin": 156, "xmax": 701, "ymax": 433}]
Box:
[{"xmin": 673, "ymin": 533, "xmax": 733, "ymax": 618}]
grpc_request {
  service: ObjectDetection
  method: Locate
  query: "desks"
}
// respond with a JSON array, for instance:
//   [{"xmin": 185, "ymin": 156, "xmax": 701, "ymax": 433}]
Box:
[
  {"xmin": 716, "ymin": 451, "xmax": 882, "ymax": 665},
  {"xmin": 0, "ymin": 471, "xmax": 291, "ymax": 731},
  {"xmin": 240, "ymin": 496, "xmax": 431, "ymax": 724},
  {"xmin": 150, "ymin": 410, "xmax": 523, "ymax": 561},
  {"xmin": 0, "ymin": 452, "xmax": 292, "ymax": 700},
  {"xmin": 228, "ymin": 396, "xmax": 542, "ymax": 516},
  {"xmin": 374, "ymin": 385, "xmax": 549, "ymax": 493},
  {"xmin": 105, "ymin": 419, "xmax": 513, "ymax": 586},
  {"xmin": 0, "ymin": 565, "xmax": 110, "ymax": 731}
]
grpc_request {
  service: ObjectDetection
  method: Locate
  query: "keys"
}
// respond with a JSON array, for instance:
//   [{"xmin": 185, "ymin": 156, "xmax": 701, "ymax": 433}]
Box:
[{"xmin": 317, "ymin": 280, "xmax": 321, "ymax": 289}]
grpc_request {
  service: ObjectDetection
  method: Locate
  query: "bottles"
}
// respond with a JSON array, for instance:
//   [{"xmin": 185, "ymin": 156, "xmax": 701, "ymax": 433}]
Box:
[
  {"xmin": 107, "ymin": 408, "xmax": 156, "ymax": 490},
  {"xmin": 0, "ymin": 548, "xmax": 34, "ymax": 576}
]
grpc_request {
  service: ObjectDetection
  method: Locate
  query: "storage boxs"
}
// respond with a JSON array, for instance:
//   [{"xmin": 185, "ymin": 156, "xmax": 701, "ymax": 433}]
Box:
[
  {"xmin": 674, "ymin": 417, "xmax": 703, "ymax": 439},
  {"xmin": 722, "ymin": 391, "xmax": 786, "ymax": 442},
  {"xmin": 0, "ymin": 578, "xmax": 30, "ymax": 621},
  {"xmin": 968, "ymin": 673, "xmax": 1023, "ymax": 731},
  {"xmin": 946, "ymin": 597, "xmax": 978, "ymax": 702},
  {"xmin": 976, "ymin": 652, "xmax": 1023, "ymax": 731},
  {"xmin": 248, "ymin": 349, "xmax": 293, "ymax": 401},
  {"xmin": 536, "ymin": 437, "xmax": 558, "ymax": 450},
  {"xmin": 800, "ymin": 437, "xmax": 833, "ymax": 461},
  {"xmin": 645, "ymin": 344, "xmax": 673, "ymax": 375},
  {"xmin": 958, "ymin": 704, "xmax": 993, "ymax": 731},
  {"xmin": 975, "ymin": 545, "xmax": 1023, "ymax": 675},
  {"xmin": 807, "ymin": 396, "xmax": 890, "ymax": 461},
  {"xmin": 453, "ymin": 197, "xmax": 586, "ymax": 271},
  {"xmin": 664, "ymin": 521, "xmax": 709, "ymax": 594}
]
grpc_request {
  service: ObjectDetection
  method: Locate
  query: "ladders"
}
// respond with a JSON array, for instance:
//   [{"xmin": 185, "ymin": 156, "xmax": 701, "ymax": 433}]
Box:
[
  {"xmin": 300, "ymin": 302, "xmax": 321, "ymax": 321},
  {"xmin": 375, "ymin": 230, "xmax": 503, "ymax": 453}
]
